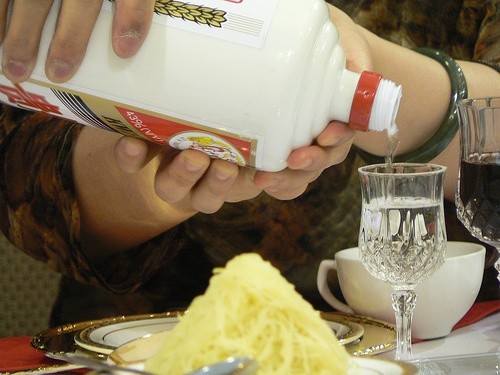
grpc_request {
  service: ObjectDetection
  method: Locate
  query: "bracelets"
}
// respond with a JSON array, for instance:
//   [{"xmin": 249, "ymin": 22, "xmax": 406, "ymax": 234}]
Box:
[{"xmin": 357, "ymin": 46, "xmax": 468, "ymax": 172}]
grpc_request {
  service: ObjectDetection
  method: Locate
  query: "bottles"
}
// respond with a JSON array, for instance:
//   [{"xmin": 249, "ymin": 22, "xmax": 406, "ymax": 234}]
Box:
[{"xmin": 0, "ymin": 0, "xmax": 401, "ymax": 173}]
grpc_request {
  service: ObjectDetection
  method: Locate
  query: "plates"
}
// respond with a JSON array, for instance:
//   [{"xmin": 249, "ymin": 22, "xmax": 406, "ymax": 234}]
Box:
[
  {"xmin": 106, "ymin": 331, "xmax": 420, "ymax": 375},
  {"xmin": 73, "ymin": 319, "xmax": 365, "ymax": 354}
]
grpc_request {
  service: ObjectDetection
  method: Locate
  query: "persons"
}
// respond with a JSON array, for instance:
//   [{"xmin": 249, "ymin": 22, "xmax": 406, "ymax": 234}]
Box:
[
  {"xmin": 0, "ymin": 0, "xmax": 500, "ymax": 330},
  {"xmin": 0, "ymin": 0, "xmax": 156, "ymax": 84}
]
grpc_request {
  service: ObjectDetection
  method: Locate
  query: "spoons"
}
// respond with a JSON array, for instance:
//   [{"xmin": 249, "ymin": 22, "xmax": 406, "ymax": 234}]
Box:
[{"xmin": 43, "ymin": 352, "xmax": 258, "ymax": 375}]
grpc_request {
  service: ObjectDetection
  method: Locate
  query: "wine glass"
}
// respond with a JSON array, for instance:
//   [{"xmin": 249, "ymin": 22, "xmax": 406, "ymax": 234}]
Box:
[
  {"xmin": 455, "ymin": 98, "xmax": 500, "ymax": 287},
  {"xmin": 357, "ymin": 161, "xmax": 447, "ymax": 363}
]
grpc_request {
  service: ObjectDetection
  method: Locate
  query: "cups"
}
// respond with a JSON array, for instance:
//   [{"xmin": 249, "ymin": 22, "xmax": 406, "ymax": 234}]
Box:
[
  {"xmin": 316, "ymin": 241, "xmax": 486, "ymax": 339},
  {"xmin": 31, "ymin": 312, "xmax": 398, "ymax": 360}
]
